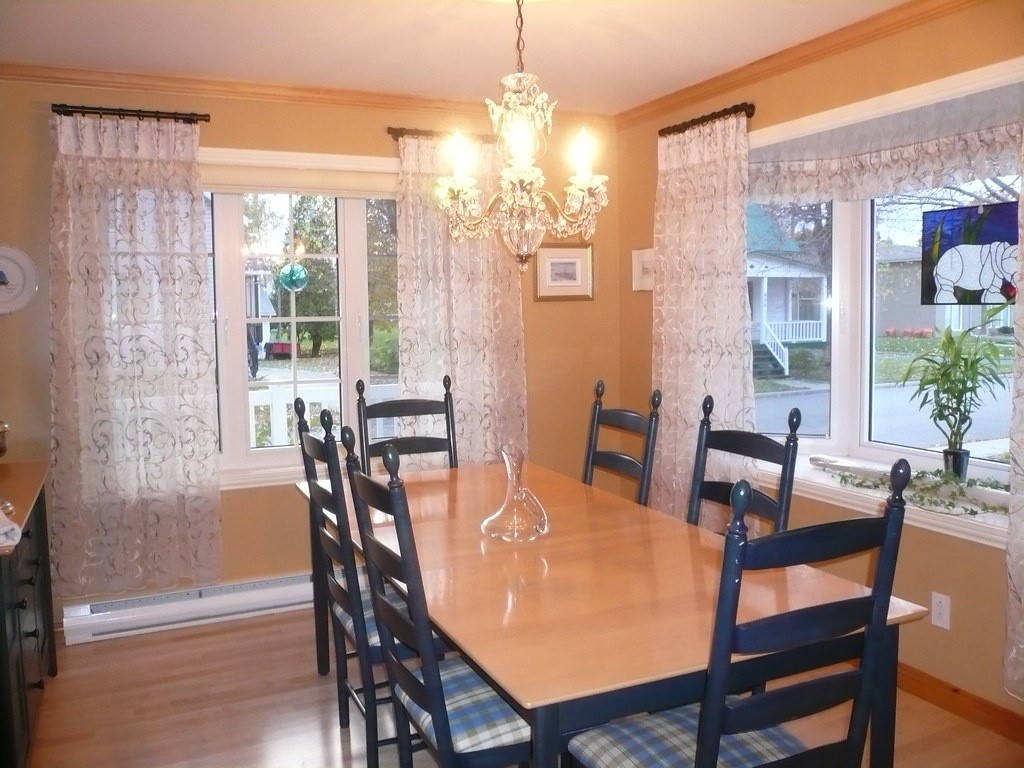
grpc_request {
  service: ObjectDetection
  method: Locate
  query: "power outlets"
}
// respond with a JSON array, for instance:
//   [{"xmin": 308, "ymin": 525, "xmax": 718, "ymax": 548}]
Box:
[{"xmin": 929, "ymin": 590, "xmax": 951, "ymax": 631}]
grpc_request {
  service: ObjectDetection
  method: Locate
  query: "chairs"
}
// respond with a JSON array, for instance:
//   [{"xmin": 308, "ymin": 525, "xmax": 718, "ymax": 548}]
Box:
[
  {"xmin": 568, "ymin": 458, "xmax": 915, "ymax": 768},
  {"xmin": 294, "ymin": 397, "xmax": 457, "ymax": 768},
  {"xmin": 339, "ymin": 425, "xmax": 568, "ymax": 768},
  {"xmin": 355, "ymin": 375, "xmax": 464, "ymax": 477},
  {"xmin": 685, "ymin": 394, "xmax": 801, "ymax": 540},
  {"xmin": 584, "ymin": 379, "xmax": 663, "ymax": 507}
]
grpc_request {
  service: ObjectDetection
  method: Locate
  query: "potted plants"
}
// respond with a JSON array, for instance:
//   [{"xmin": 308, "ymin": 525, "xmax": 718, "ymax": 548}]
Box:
[{"xmin": 899, "ymin": 296, "xmax": 1016, "ymax": 483}]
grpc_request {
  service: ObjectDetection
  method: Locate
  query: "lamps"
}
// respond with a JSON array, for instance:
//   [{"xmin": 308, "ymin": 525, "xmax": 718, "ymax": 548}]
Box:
[{"xmin": 431, "ymin": 0, "xmax": 608, "ymax": 270}]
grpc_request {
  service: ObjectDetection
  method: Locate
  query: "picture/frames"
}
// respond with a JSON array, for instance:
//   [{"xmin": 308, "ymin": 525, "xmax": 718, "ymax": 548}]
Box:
[
  {"xmin": 533, "ymin": 242, "xmax": 595, "ymax": 301},
  {"xmin": 630, "ymin": 248, "xmax": 656, "ymax": 293}
]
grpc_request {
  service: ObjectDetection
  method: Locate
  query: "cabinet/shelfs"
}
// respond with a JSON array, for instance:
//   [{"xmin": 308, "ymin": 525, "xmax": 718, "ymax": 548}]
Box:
[{"xmin": 0, "ymin": 485, "xmax": 59, "ymax": 768}]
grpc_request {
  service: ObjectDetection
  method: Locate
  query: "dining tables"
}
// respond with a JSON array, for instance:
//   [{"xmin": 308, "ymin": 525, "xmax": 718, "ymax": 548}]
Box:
[{"xmin": 290, "ymin": 459, "xmax": 930, "ymax": 767}]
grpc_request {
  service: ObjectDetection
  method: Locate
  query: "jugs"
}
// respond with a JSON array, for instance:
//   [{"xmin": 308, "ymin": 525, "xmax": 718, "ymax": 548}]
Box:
[{"xmin": 477, "ymin": 442, "xmax": 550, "ymax": 544}]
